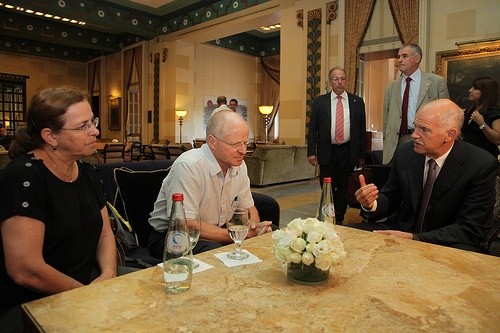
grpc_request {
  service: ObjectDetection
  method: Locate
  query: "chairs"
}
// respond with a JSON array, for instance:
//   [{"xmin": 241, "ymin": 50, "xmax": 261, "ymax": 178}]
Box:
[
  {"xmin": 85, "ymin": 158, "xmax": 281, "ymax": 269},
  {"xmin": 97, "ymin": 139, "xmax": 207, "ymax": 163}
]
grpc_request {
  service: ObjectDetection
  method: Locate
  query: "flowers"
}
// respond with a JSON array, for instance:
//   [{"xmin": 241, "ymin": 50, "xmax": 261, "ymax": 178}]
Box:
[{"xmin": 271, "ymin": 216, "xmax": 349, "ymax": 273}]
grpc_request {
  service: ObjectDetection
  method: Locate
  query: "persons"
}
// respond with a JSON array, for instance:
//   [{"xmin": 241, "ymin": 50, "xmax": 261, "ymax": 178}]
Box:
[
  {"xmin": 210, "ymin": 96, "xmax": 232, "ymax": 116},
  {"xmin": 462, "ymin": 77, "xmax": 500, "ymax": 158},
  {"xmin": 145, "ymin": 110, "xmax": 272, "ymax": 258},
  {"xmin": 230, "ymin": 99, "xmax": 238, "ymax": 112},
  {"xmin": 8, "ymin": 127, "xmax": 28, "ymax": 160},
  {"xmin": 382, "ymin": 43, "xmax": 450, "ymax": 163},
  {"xmin": 306, "ymin": 68, "xmax": 367, "ymax": 224},
  {"xmin": 355, "ymin": 98, "xmax": 497, "ymax": 251},
  {"xmin": 0, "ymin": 87, "xmax": 117, "ymax": 333},
  {"xmin": 0, "ymin": 124, "xmax": 15, "ymax": 150}
]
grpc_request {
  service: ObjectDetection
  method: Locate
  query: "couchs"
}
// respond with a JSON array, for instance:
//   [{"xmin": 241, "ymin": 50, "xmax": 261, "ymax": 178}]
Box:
[{"xmin": 243, "ymin": 144, "xmax": 321, "ymax": 186}]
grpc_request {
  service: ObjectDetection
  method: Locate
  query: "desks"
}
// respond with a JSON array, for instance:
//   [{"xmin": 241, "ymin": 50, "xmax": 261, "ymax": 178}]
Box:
[{"xmin": 21, "ymin": 219, "xmax": 500, "ymax": 333}]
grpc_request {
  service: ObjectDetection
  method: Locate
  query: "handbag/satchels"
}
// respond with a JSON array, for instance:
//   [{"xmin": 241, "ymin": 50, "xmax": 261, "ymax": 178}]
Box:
[{"xmin": 106, "ymin": 202, "xmax": 138, "ymax": 256}]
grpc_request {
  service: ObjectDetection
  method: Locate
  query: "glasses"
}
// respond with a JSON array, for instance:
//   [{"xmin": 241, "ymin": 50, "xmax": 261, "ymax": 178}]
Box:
[
  {"xmin": 59, "ymin": 117, "xmax": 99, "ymax": 134},
  {"xmin": 212, "ymin": 134, "xmax": 251, "ymax": 149}
]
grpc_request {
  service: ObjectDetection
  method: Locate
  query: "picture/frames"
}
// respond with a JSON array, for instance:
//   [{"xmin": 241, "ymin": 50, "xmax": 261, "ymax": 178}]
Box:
[{"xmin": 108, "ymin": 97, "xmax": 121, "ymax": 132}]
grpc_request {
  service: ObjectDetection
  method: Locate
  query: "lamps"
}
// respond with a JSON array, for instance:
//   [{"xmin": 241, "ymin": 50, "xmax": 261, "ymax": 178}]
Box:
[
  {"xmin": 258, "ymin": 105, "xmax": 273, "ymax": 142},
  {"xmin": 258, "ymin": 48, "xmax": 265, "ymax": 56},
  {"xmin": 175, "ymin": 110, "xmax": 188, "ymax": 143}
]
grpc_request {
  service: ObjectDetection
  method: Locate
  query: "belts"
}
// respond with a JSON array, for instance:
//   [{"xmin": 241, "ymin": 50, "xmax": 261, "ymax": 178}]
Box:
[{"xmin": 408, "ymin": 129, "xmax": 415, "ymax": 134}]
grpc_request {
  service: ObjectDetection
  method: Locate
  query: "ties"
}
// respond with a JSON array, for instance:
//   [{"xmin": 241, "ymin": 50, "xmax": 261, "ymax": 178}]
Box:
[
  {"xmin": 335, "ymin": 95, "xmax": 344, "ymax": 144},
  {"xmin": 401, "ymin": 78, "xmax": 413, "ymax": 136},
  {"xmin": 417, "ymin": 160, "xmax": 437, "ymax": 232}
]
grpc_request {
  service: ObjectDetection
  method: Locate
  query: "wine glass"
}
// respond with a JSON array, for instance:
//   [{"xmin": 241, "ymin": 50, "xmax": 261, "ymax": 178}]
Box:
[
  {"xmin": 183, "ymin": 211, "xmax": 201, "ymax": 270},
  {"xmin": 226, "ymin": 207, "xmax": 251, "ymax": 260}
]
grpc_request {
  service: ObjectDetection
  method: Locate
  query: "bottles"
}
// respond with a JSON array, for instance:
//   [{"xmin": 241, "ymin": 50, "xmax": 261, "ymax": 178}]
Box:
[
  {"xmin": 316, "ymin": 177, "xmax": 335, "ymax": 227},
  {"xmin": 163, "ymin": 193, "xmax": 193, "ymax": 294}
]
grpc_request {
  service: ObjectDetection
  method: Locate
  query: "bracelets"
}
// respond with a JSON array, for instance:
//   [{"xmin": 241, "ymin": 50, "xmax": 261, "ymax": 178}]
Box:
[{"xmin": 479, "ymin": 125, "xmax": 485, "ymax": 129}]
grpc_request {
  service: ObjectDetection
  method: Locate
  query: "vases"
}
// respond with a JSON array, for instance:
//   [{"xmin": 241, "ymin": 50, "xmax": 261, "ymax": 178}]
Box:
[{"xmin": 285, "ymin": 259, "xmax": 331, "ymax": 284}]
